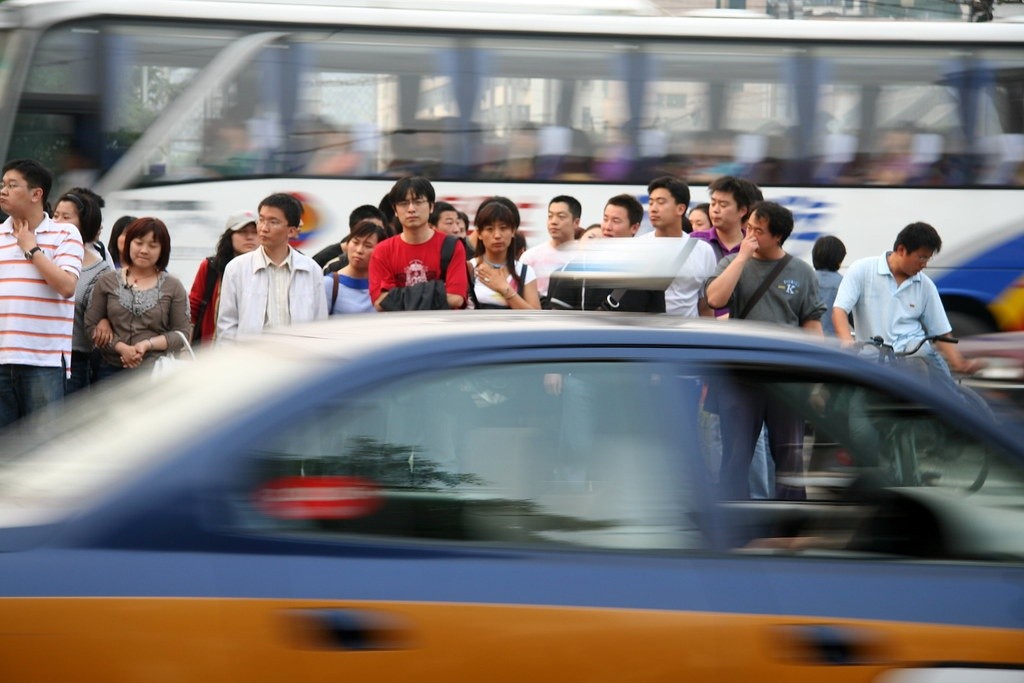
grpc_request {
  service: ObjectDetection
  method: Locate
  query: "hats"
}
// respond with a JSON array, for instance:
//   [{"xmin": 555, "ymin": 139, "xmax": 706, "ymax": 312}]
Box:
[{"xmin": 226, "ymin": 212, "xmax": 257, "ymax": 231}]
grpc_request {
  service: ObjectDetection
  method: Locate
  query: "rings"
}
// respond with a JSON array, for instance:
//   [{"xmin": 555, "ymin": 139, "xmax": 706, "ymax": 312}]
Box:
[{"xmin": 484, "ymin": 277, "xmax": 489, "ymax": 282}]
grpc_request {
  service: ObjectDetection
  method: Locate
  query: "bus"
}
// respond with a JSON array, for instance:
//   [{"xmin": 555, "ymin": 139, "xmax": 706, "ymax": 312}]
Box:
[{"xmin": 0, "ymin": 0, "xmax": 1024, "ymax": 403}]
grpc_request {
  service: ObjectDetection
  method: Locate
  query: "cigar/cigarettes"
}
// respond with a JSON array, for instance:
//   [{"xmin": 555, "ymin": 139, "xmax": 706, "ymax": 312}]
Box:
[{"xmin": 819, "ymin": 412, "xmax": 825, "ymax": 418}]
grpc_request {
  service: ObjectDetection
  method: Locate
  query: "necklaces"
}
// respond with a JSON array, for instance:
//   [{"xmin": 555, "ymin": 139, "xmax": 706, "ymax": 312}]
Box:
[
  {"xmin": 482, "ymin": 258, "xmax": 507, "ymax": 269},
  {"xmin": 130, "ymin": 265, "xmax": 156, "ymax": 288}
]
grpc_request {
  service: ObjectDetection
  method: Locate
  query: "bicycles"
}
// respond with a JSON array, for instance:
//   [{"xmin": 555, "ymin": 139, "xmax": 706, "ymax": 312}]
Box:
[{"xmin": 844, "ymin": 326, "xmax": 988, "ymax": 491}]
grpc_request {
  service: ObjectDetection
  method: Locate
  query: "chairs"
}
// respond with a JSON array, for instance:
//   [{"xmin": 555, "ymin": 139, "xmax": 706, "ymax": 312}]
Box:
[{"xmin": 244, "ymin": 111, "xmax": 1024, "ymax": 187}]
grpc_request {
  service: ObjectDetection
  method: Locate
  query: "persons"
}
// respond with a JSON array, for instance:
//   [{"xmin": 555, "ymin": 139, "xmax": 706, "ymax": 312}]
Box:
[
  {"xmin": 55, "ymin": 110, "xmax": 990, "ymax": 188},
  {"xmin": 49, "ymin": 175, "xmax": 989, "ymax": 507},
  {"xmin": 0, "ymin": 160, "xmax": 84, "ymax": 438}
]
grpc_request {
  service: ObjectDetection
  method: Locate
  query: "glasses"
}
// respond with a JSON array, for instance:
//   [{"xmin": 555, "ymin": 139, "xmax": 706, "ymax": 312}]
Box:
[
  {"xmin": 398, "ymin": 199, "xmax": 429, "ymax": 207},
  {"xmin": 0, "ymin": 181, "xmax": 37, "ymax": 190},
  {"xmin": 912, "ymin": 252, "xmax": 935, "ymax": 265}
]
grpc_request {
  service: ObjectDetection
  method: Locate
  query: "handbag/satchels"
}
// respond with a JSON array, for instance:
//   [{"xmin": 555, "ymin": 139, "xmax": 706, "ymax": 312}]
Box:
[{"xmin": 151, "ymin": 331, "xmax": 196, "ymax": 379}]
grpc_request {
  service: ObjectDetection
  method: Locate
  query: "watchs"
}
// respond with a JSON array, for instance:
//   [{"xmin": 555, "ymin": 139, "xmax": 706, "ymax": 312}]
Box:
[{"xmin": 25, "ymin": 247, "xmax": 44, "ymax": 263}]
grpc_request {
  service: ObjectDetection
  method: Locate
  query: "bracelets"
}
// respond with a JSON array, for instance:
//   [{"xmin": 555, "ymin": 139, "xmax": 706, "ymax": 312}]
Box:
[
  {"xmin": 502, "ymin": 283, "xmax": 510, "ymax": 296},
  {"xmin": 148, "ymin": 337, "xmax": 153, "ymax": 351},
  {"xmin": 505, "ymin": 291, "xmax": 517, "ymax": 300},
  {"xmin": 810, "ymin": 389, "xmax": 820, "ymax": 394}
]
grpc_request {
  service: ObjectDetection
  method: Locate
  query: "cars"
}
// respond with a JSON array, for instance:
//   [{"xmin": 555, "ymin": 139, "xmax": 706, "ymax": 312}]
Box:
[{"xmin": 0, "ymin": 311, "xmax": 1024, "ymax": 683}]
